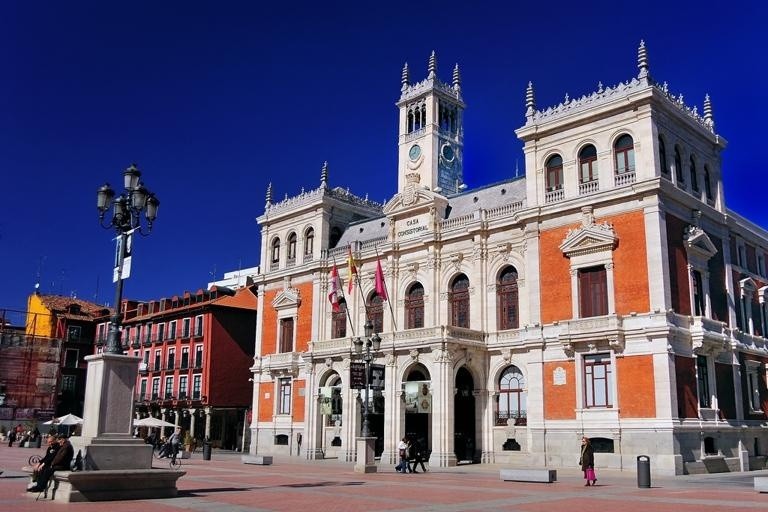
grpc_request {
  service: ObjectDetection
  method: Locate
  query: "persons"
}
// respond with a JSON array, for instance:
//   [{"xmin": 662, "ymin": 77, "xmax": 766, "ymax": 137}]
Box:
[
  {"xmin": 399, "ymin": 438, "xmax": 408, "ymax": 473},
  {"xmin": 135, "ymin": 426, "xmax": 158, "ymax": 449},
  {"xmin": 124, "ymin": 234, "xmax": 133, "ymax": 256},
  {"xmin": 7, "ymin": 423, "xmax": 40, "ymax": 448},
  {"xmin": 167, "ymin": 427, "xmax": 182, "ymax": 465},
  {"xmin": 26, "ymin": 437, "xmax": 74, "ymax": 492},
  {"xmin": 395, "ymin": 439, "xmax": 412, "ymax": 473},
  {"xmin": 158, "ymin": 432, "xmax": 170, "ymax": 458},
  {"xmin": 48, "ymin": 426, "xmax": 56, "ymax": 436},
  {"xmin": 413, "ymin": 436, "xmax": 430, "ymax": 474},
  {"xmin": 115, "ymin": 239, "xmax": 121, "ymax": 267},
  {"xmin": 26, "ymin": 437, "xmax": 60, "ymax": 492},
  {"xmin": 579, "ymin": 436, "xmax": 598, "ymax": 487}
]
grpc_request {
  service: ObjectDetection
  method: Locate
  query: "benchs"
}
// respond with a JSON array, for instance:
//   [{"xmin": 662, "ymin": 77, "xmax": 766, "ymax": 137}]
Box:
[
  {"xmin": 241, "ymin": 455, "xmax": 273, "ymax": 465},
  {"xmin": 499, "ymin": 468, "xmax": 557, "ymax": 485},
  {"xmin": 169, "ymin": 449, "xmax": 191, "ymax": 459}
]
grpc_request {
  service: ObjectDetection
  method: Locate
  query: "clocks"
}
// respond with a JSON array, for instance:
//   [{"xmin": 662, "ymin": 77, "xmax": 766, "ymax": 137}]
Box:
[
  {"xmin": 441, "ymin": 144, "xmax": 455, "ymax": 162},
  {"xmin": 409, "ymin": 144, "xmax": 422, "ymax": 160}
]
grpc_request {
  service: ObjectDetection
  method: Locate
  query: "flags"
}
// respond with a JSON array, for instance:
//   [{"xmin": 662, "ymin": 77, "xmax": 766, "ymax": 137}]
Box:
[
  {"xmin": 347, "ymin": 249, "xmax": 357, "ymax": 295},
  {"xmin": 328, "ymin": 262, "xmax": 345, "ymax": 314},
  {"xmin": 375, "ymin": 258, "xmax": 392, "ymax": 300}
]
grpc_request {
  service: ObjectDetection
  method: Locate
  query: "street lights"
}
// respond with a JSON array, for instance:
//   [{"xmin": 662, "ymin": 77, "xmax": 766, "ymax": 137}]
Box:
[
  {"xmin": 354, "ymin": 331, "xmax": 382, "ymax": 438},
  {"xmin": 94, "ymin": 162, "xmax": 160, "ymax": 356}
]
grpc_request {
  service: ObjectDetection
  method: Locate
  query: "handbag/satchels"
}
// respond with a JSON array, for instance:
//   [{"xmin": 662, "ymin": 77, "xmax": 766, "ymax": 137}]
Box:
[{"xmin": 586, "ymin": 467, "xmax": 596, "ymax": 480}]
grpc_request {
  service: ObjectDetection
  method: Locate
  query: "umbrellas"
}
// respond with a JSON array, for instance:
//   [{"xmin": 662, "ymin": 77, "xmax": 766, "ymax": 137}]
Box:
[
  {"xmin": 133, "ymin": 413, "xmax": 182, "ymax": 429},
  {"xmin": 42, "ymin": 411, "xmax": 86, "ymax": 435}
]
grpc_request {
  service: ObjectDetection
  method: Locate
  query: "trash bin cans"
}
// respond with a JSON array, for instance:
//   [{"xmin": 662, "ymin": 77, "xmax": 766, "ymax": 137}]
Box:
[
  {"xmin": 637, "ymin": 455, "xmax": 650, "ymax": 488},
  {"xmin": 203, "ymin": 442, "xmax": 212, "ymax": 460}
]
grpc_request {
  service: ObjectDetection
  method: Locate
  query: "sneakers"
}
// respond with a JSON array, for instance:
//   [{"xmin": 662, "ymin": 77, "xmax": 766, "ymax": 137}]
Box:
[
  {"xmin": 584, "ymin": 479, "xmax": 597, "ymax": 486},
  {"xmin": 26, "ymin": 485, "xmax": 48, "ymax": 493}
]
